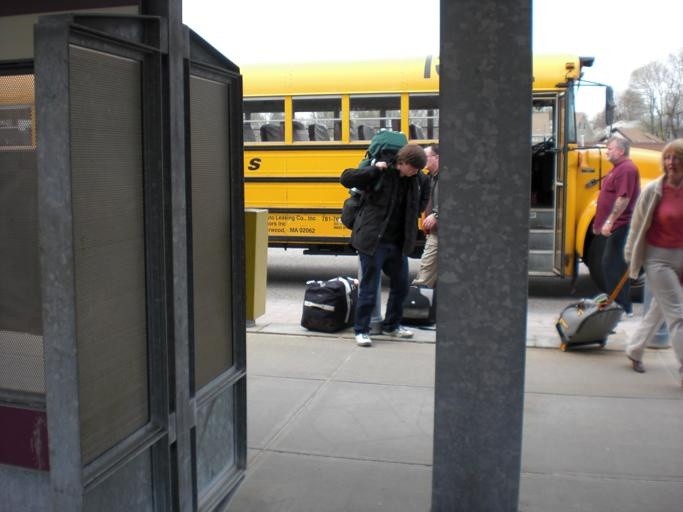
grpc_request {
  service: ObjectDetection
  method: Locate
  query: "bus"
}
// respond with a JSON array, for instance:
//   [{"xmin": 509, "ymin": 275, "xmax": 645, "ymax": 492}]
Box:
[{"xmin": 2, "ymin": 57, "xmax": 666, "ymax": 304}]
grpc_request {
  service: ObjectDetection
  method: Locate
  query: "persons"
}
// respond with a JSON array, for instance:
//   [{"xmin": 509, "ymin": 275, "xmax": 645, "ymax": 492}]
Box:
[
  {"xmin": 412, "ymin": 144, "xmax": 446, "ymax": 286},
  {"xmin": 592, "ymin": 136, "xmax": 641, "ymax": 322},
  {"xmin": 339, "ymin": 143, "xmax": 431, "ymax": 345},
  {"xmin": 623, "ymin": 138, "xmax": 683, "ymax": 373}
]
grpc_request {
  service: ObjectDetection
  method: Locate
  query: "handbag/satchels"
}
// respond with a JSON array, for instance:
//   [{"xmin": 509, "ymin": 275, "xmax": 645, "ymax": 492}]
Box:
[
  {"xmin": 400, "ymin": 284, "xmax": 436, "ymax": 326},
  {"xmin": 301, "ymin": 275, "xmax": 359, "ymax": 334}
]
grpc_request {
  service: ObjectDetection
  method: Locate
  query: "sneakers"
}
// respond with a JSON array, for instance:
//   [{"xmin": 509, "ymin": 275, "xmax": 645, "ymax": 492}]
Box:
[
  {"xmin": 382, "ymin": 325, "xmax": 413, "ymax": 337},
  {"xmin": 355, "ymin": 333, "xmax": 372, "ymax": 346},
  {"xmin": 628, "ymin": 353, "xmax": 644, "ymax": 372}
]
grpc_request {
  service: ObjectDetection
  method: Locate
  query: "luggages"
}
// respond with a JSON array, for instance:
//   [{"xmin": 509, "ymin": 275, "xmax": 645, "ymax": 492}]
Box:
[{"xmin": 556, "ymin": 268, "xmax": 628, "ymax": 351}]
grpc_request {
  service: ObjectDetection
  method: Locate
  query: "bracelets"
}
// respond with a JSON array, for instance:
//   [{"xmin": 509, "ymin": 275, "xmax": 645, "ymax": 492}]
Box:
[
  {"xmin": 433, "ymin": 212, "xmax": 439, "ymax": 219},
  {"xmin": 605, "ymin": 220, "xmax": 611, "ymax": 224}
]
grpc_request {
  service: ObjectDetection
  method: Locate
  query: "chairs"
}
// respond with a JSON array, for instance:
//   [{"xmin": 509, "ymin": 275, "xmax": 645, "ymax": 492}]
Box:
[{"xmin": 241, "ymin": 116, "xmax": 427, "ymax": 141}]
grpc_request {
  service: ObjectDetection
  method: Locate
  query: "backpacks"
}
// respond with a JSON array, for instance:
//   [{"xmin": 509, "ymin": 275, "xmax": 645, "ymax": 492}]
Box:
[{"xmin": 341, "ymin": 128, "xmax": 408, "ymax": 230}]
